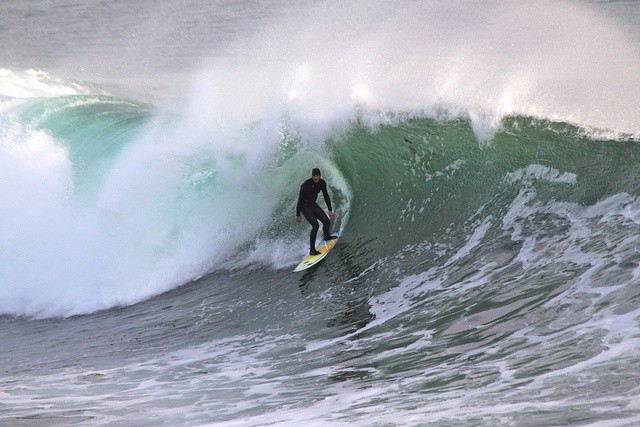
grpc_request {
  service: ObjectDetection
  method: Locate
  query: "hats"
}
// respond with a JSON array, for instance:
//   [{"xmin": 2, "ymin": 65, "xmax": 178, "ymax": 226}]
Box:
[{"xmin": 312, "ymin": 168, "xmax": 322, "ymax": 177}]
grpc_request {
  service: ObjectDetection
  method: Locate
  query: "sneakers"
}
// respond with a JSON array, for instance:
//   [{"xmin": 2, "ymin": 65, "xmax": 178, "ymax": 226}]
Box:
[
  {"xmin": 309, "ymin": 248, "xmax": 321, "ymax": 256},
  {"xmin": 322, "ymin": 235, "xmax": 339, "ymax": 242}
]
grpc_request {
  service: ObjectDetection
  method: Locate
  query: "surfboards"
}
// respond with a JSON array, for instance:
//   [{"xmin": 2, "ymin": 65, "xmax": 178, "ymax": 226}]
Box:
[{"xmin": 293, "ymin": 232, "xmax": 339, "ymax": 272}]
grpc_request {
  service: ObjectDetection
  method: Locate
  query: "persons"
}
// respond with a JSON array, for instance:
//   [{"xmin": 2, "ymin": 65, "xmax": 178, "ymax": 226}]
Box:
[{"xmin": 296, "ymin": 168, "xmax": 338, "ymax": 255}]
